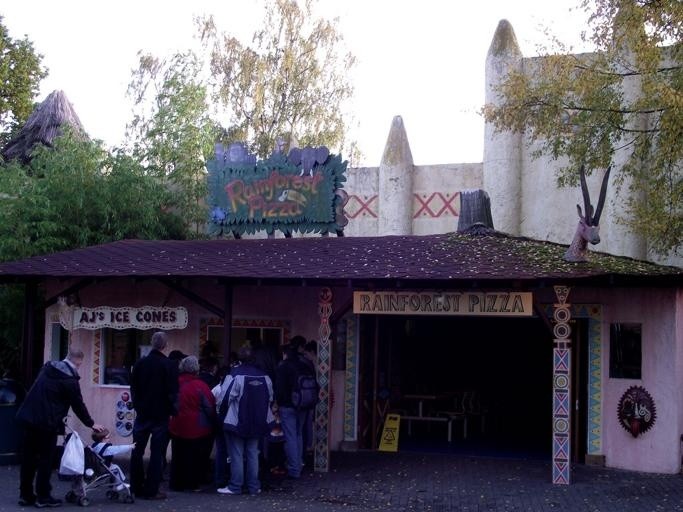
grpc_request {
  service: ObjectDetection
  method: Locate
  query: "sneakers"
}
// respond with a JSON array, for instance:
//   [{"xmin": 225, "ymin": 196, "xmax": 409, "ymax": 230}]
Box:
[
  {"xmin": 217, "ymin": 485, "xmax": 262, "ymax": 496},
  {"xmin": 18, "ymin": 496, "xmax": 65, "ymax": 508}
]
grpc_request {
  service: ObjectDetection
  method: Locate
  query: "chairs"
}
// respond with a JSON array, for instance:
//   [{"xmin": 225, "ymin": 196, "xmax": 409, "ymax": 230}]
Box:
[{"xmin": 362, "ymin": 390, "xmax": 489, "ymax": 442}]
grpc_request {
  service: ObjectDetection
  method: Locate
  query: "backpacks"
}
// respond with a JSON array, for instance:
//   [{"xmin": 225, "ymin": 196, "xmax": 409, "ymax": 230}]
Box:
[{"xmin": 291, "ymin": 368, "xmax": 321, "ymax": 410}]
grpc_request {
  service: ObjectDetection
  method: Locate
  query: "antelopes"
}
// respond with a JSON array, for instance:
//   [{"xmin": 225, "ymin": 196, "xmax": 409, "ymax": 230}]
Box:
[{"xmin": 560, "ymin": 161, "xmax": 612, "ymax": 263}]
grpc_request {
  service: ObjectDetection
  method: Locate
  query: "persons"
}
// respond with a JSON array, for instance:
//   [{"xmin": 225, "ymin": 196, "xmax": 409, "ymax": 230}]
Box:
[
  {"xmin": 168, "ymin": 333, "xmax": 334, "ymax": 494},
  {"xmin": 126, "ymin": 331, "xmax": 179, "ymax": 500},
  {"xmin": 89, "ymin": 427, "xmax": 137, "ymax": 491},
  {"xmin": 15, "ymin": 346, "xmax": 106, "ymax": 507}
]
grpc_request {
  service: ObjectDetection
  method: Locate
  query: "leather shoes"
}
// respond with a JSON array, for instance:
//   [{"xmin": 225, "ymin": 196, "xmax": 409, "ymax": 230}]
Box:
[{"xmin": 143, "ymin": 492, "xmax": 168, "ymax": 500}]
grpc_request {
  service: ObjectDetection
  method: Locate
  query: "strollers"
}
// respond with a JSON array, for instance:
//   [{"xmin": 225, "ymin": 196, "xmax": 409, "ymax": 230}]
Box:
[{"xmin": 62, "ymin": 416, "xmax": 135, "ymax": 506}]
